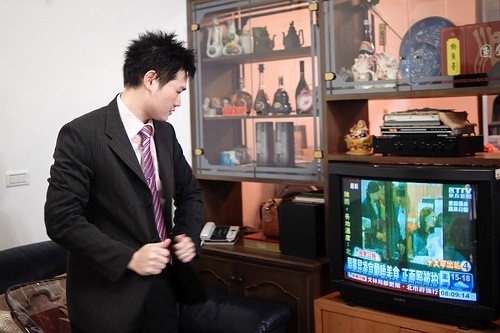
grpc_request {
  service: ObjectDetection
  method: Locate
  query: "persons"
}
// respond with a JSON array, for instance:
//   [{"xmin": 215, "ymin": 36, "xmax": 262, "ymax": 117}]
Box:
[
  {"xmin": 44, "ymin": 30, "xmax": 204, "ymax": 333},
  {"xmin": 350, "ymin": 120, "xmax": 369, "ymax": 138},
  {"xmin": 361, "ymin": 182, "xmax": 434, "ymax": 262}
]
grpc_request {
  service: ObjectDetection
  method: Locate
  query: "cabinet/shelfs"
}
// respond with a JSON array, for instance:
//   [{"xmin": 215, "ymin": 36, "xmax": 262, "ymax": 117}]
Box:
[{"xmin": 185, "ymin": 0, "xmax": 500, "ymax": 333}]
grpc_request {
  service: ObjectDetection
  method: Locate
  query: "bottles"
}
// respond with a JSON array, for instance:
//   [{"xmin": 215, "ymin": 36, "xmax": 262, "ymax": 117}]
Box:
[
  {"xmin": 295, "ymin": 60, "xmax": 312, "ymax": 115},
  {"xmin": 254, "ymin": 63, "xmax": 270, "ymax": 116},
  {"xmin": 270, "ymin": 76, "xmax": 292, "ymax": 116},
  {"xmin": 358, "ymin": 19, "xmax": 376, "ymax": 81},
  {"xmin": 234, "ymin": 77, "xmax": 252, "ymax": 116}
]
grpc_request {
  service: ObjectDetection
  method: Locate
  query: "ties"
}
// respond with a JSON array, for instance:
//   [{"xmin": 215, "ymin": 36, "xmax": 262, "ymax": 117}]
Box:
[{"xmin": 138, "ymin": 125, "xmax": 173, "ymax": 264}]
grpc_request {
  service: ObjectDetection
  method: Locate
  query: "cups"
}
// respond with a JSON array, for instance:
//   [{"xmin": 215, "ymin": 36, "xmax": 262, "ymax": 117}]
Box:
[
  {"xmin": 218, "ymin": 151, "xmax": 237, "ymax": 167},
  {"xmin": 239, "ymin": 35, "xmax": 251, "ymax": 54}
]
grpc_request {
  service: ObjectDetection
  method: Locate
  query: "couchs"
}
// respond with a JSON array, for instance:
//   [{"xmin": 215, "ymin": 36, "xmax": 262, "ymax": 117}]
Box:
[{"xmin": 0, "ymin": 239, "xmax": 295, "ymax": 333}]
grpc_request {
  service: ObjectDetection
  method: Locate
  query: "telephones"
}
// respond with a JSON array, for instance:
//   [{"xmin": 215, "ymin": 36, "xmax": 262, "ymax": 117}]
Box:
[{"xmin": 199, "ymin": 222, "xmax": 242, "ymax": 244}]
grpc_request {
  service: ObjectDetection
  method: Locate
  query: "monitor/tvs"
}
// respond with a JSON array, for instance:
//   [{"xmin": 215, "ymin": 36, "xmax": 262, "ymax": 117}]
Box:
[{"xmin": 328, "ymin": 157, "xmax": 500, "ymax": 330}]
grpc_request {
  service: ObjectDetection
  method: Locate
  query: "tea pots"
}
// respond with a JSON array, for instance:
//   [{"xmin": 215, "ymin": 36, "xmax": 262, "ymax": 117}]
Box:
[
  {"xmin": 254, "ymin": 31, "xmax": 276, "ymax": 54},
  {"xmin": 281, "ymin": 21, "xmax": 304, "ymax": 49}
]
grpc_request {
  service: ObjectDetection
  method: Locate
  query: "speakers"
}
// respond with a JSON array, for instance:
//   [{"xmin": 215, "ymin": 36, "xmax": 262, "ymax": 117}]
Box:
[{"xmin": 278, "ymin": 189, "xmax": 325, "ymax": 258}]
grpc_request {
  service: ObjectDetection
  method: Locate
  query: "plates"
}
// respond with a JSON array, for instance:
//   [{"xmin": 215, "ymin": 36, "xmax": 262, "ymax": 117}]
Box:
[
  {"xmin": 399, "ymin": 43, "xmax": 441, "ymax": 86},
  {"xmin": 399, "ymin": 16, "xmax": 457, "ymax": 59}
]
